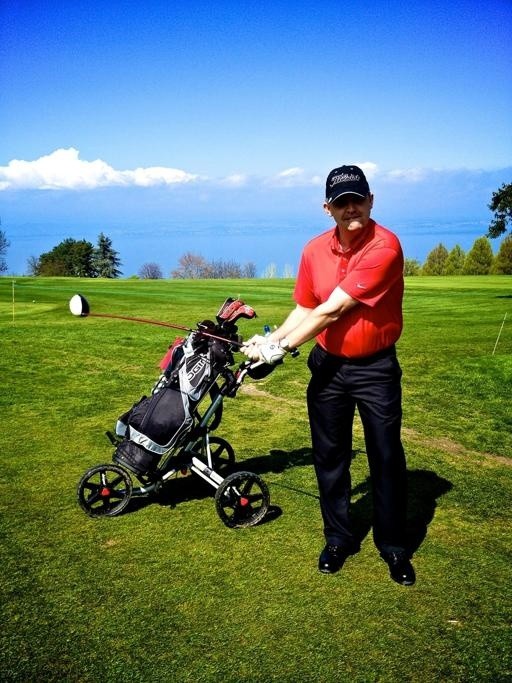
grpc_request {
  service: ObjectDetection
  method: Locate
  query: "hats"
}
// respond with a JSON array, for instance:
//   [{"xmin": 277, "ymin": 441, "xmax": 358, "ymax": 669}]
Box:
[{"xmin": 326, "ymin": 165, "xmax": 369, "ymax": 204}]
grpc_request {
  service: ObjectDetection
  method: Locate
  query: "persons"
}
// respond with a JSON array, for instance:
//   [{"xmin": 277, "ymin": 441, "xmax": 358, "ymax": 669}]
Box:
[{"xmin": 238, "ymin": 164, "xmax": 418, "ymax": 586}]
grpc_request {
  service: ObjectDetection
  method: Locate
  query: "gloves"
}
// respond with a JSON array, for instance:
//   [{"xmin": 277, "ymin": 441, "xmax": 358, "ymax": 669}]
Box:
[{"xmin": 259, "ymin": 340, "xmax": 286, "ymax": 365}]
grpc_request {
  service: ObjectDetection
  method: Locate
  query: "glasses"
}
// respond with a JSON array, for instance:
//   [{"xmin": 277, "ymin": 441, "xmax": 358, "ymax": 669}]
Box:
[{"xmin": 333, "ymin": 196, "xmax": 366, "ymax": 209}]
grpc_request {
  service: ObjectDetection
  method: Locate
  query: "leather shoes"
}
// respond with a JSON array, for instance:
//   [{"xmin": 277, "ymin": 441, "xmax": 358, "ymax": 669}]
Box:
[
  {"xmin": 380, "ymin": 551, "xmax": 416, "ymax": 586},
  {"xmin": 319, "ymin": 544, "xmax": 346, "ymax": 574}
]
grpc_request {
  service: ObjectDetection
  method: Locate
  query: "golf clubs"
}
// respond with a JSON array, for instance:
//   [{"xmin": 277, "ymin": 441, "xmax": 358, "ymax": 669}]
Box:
[
  {"xmin": 217, "ymin": 297, "xmax": 257, "ymax": 329},
  {"xmin": 69, "ymin": 294, "xmax": 247, "ymax": 347}
]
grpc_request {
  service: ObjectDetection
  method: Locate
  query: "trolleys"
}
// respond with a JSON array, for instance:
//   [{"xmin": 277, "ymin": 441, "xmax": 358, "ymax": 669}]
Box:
[{"xmin": 77, "ymin": 317, "xmax": 275, "ymax": 529}]
